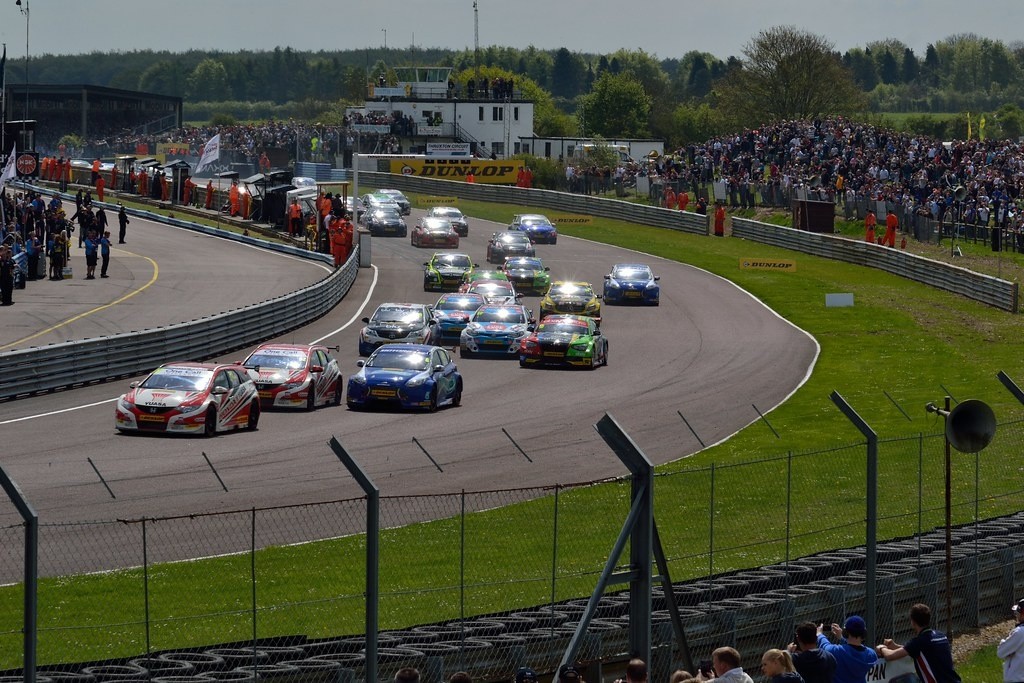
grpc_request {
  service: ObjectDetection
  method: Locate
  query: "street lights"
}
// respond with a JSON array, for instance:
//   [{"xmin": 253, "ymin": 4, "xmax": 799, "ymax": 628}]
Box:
[{"xmin": 452, "ymin": 97, "xmax": 458, "ymax": 141}]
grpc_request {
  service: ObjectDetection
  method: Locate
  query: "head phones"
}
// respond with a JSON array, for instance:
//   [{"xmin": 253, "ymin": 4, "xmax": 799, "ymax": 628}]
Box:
[{"xmin": 842, "ymin": 624, "xmax": 867, "ymax": 639}]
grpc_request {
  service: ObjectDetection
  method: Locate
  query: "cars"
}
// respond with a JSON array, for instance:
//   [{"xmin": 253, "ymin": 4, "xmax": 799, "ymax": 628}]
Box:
[
  {"xmin": 520, "ymin": 313, "xmax": 609, "ymax": 369},
  {"xmin": 291, "ymin": 176, "xmax": 325, "ymax": 193},
  {"xmin": 410, "ymin": 215, "xmax": 459, "ymax": 249},
  {"xmin": 433, "ymin": 291, "xmax": 489, "ymax": 341},
  {"xmin": 70, "ymin": 159, "xmax": 118, "ymax": 170},
  {"xmin": 458, "ymin": 302, "xmax": 536, "ymax": 357},
  {"xmin": 358, "ymin": 302, "xmax": 439, "ymax": 355},
  {"xmin": 237, "ymin": 342, "xmax": 344, "ymax": 411},
  {"xmin": 360, "ymin": 207, "xmax": 407, "ymax": 237},
  {"xmin": 540, "ymin": 280, "xmax": 603, "ymax": 325},
  {"xmin": 508, "ymin": 213, "xmax": 557, "ymax": 244},
  {"xmin": 497, "ymin": 256, "xmax": 550, "ymax": 293},
  {"xmin": 603, "ymin": 263, "xmax": 661, "ymax": 305},
  {"xmin": 424, "ymin": 251, "xmax": 479, "ymax": 292},
  {"xmin": 487, "ymin": 229, "xmax": 536, "ymax": 263},
  {"xmin": 341, "ymin": 187, "xmax": 411, "ymax": 218},
  {"xmin": 348, "ymin": 344, "xmax": 463, "ymax": 412},
  {"xmin": 116, "ymin": 362, "xmax": 263, "ymax": 436},
  {"xmin": 459, "ymin": 270, "xmax": 525, "ymax": 306},
  {"xmin": 428, "ymin": 207, "xmax": 469, "ymax": 237}
]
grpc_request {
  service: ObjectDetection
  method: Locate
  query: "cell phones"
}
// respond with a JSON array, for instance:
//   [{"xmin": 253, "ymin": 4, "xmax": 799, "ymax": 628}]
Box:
[
  {"xmin": 822, "ymin": 626, "xmax": 831, "ymax": 631},
  {"xmin": 700, "ymin": 660, "xmax": 713, "ymax": 677},
  {"xmin": 793, "ymin": 634, "xmax": 801, "ymax": 651}
]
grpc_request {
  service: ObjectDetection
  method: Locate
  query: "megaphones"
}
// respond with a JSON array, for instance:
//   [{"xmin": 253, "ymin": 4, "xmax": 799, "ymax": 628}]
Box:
[{"xmin": 924, "ymin": 399, "xmax": 997, "ymax": 453}]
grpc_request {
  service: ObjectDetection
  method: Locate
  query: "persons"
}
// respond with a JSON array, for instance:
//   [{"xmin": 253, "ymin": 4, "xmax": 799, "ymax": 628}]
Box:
[
  {"xmin": 995, "ymin": 598, "xmax": 1024, "ymax": 683},
  {"xmin": 449, "ymin": 670, "xmax": 472, "ymax": 683},
  {"xmin": 558, "ymin": 663, "xmax": 586, "ymax": 683},
  {"xmin": 881, "ymin": 208, "xmax": 899, "ymax": 250},
  {"xmin": 0, "ymin": 183, "xmax": 129, "ymax": 308},
  {"xmin": 465, "ymin": 112, "xmax": 1024, "ymax": 270},
  {"xmin": 866, "ymin": 207, "xmax": 878, "ymax": 244},
  {"xmin": 447, "ymin": 75, "xmax": 515, "ymax": 102},
  {"xmin": 392, "ymin": 667, "xmax": 421, "ymax": 683},
  {"xmin": 875, "ymin": 604, "xmax": 958, "ymax": 683},
  {"xmin": 669, "ymin": 613, "xmax": 879, "ymax": 683},
  {"xmin": 15, "ymin": 107, "xmax": 444, "ymax": 269},
  {"xmin": 512, "ymin": 665, "xmax": 538, "ymax": 683},
  {"xmin": 613, "ymin": 657, "xmax": 653, "ymax": 683}
]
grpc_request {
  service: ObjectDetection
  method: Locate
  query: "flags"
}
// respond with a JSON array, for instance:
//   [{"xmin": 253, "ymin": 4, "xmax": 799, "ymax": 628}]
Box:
[
  {"xmin": 195, "ymin": 132, "xmax": 221, "ymax": 172},
  {"xmin": 0, "ymin": 146, "xmax": 15, "ymax": 193}
]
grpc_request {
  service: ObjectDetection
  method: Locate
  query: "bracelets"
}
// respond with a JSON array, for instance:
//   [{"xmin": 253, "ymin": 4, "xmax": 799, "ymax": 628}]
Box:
[{"xmin": 879, "ymin": 646, "xmax": 887, "ymax": 652}]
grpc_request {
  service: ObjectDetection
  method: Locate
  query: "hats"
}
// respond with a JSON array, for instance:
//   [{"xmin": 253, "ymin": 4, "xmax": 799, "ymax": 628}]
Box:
[
  {"xmin": 844, "ymin": 615, "xmax": 869, "ymax": 638},
  {"xmin": 1011, "ymin": 599, "xmax": 1024, "ymax": 611},
  {"xmin": 559, "ymin": 663, "xmax": 580, "ymax": 678},
  {"xmin": 516, "ymin": 669, "xmax": 537, "ymax": 683}
]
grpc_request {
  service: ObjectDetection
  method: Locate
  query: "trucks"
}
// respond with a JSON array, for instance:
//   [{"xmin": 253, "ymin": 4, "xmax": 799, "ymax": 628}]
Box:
[{"xmin": 573, "ymin": 144, "xmax": 638, "ymax": 165}]
